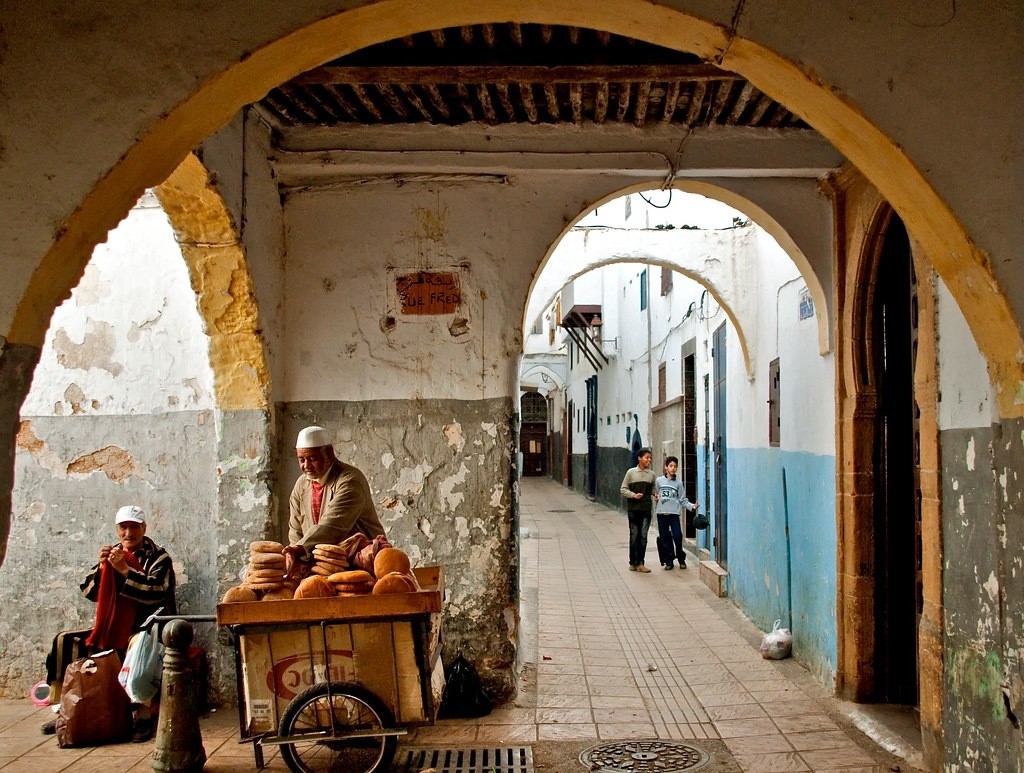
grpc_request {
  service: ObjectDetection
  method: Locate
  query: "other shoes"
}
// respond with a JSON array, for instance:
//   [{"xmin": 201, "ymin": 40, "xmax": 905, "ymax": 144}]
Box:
[
  {"xmin": 637, "ymin": 566, "xmax": 651, "ymax": 573},
  {"xmin": 41, "ymin": 715, "xmax": 62, "ymax": 734},
  {"xmin": 132, "ymin": 718, "xmax": 155, "ymax": 742},
  {"xmin": 665, "ymin": 563, "xmax": 674, "ymax": 570},
  {"xmin": 629, "ymin": 565, "xmax": 637, "ymax": 571},
  {"xmin": 680, "ymin": 563, "xmax": 686, "ymax": 569}
]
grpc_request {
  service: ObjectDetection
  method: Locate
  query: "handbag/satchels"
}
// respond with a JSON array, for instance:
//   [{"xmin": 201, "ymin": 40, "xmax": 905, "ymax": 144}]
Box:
[
  {"xmin": 118, "ymin": 615, "xmax": 166, "ymax": 704},
  {"xmin": 55, "ymin": 649, "xmax": 133, "ymax": 748}
]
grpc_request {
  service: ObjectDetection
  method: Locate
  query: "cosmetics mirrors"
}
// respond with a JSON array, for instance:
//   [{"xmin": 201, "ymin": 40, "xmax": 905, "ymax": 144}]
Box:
[{"xmin": 31, "ymin": 680, "xmax": 51, "ymax": 706}]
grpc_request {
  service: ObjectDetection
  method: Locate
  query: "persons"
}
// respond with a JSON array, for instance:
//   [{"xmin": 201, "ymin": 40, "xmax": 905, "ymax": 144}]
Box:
[
  {"xmin": 280, "ymin": 425, "xmax": 388, "ymax": 580},
  {"xmin": 41, "ymin": 505, "xmax": 176, "ymax": 735},
  {"xmin": 620, "ymin": 449, "xmax": 660, "ymax": 573},
  {"xmin": 655, "ymin": 456, "xmax": 701, "ymax": 570}
]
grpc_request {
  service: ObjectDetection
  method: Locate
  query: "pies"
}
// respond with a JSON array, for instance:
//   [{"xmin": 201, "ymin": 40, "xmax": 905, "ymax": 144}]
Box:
[{"xmin": 223, "ymin": 541, "xmax": 416, "ymax": 600}]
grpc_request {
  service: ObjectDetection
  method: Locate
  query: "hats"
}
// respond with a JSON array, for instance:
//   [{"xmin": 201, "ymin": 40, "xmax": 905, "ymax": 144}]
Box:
[
  {"xmin": 296, "ymin": 426, "xmax": 332, "ymax": 448},
  {"xmin": 115, "ymin": 506, "xmax": 146, "ymax": 525}
]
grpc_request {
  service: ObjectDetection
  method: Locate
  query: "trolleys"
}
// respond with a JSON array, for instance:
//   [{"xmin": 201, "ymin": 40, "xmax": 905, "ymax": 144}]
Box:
[{"xmin": 139, "ymin": 561, "xmax": 446, "ymax": 773}]
